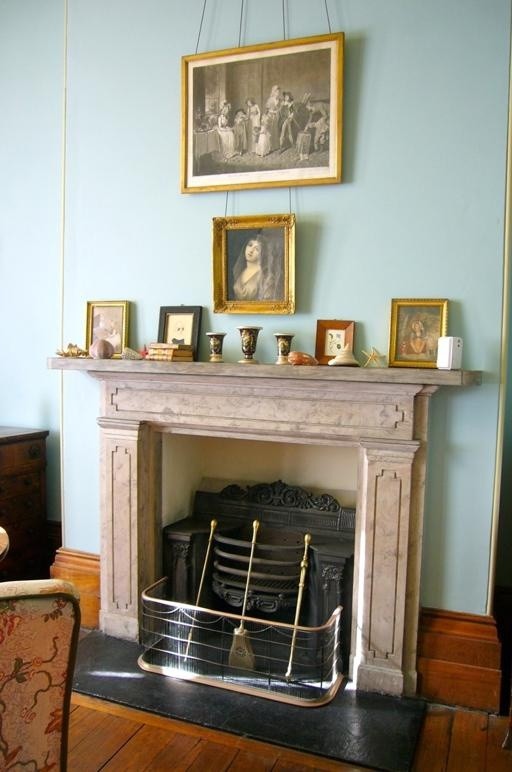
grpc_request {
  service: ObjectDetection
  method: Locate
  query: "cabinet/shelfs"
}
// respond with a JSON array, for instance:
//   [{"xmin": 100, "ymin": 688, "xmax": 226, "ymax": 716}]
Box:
[{"xmin": 0, "ymin": 426, "xmax": 48, "ymax": 574}]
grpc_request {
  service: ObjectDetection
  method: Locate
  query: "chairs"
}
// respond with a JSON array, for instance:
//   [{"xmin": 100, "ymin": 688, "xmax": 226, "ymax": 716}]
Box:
[{"xmin": 0, "ymin": 577, "xmax": 80, "ymax": 772}]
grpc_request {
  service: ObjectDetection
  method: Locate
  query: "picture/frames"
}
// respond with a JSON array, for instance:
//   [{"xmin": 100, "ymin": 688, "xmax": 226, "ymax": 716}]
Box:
[
  {"xmin": 209, "ymin": 214, "xmax": 294, "ymax": 314},
  {"xmin": 178, "ymin": 30, "xmax": 346, "ymax": 195}
]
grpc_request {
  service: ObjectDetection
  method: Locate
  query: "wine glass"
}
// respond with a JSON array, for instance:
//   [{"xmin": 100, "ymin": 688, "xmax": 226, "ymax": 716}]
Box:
[{"xmin": 236, "ymin": 326, "xmax": 261, "ymax": 364}]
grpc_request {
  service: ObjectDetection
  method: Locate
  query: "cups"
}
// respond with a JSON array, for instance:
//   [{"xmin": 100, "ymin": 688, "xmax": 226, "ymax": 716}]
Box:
[
  {"xmin": 206, "ymin": 331, "xmax": 228, "ymax": 362},
  {"xmin": 275, "ymin": 334, "xmax": 293, "ymax": 363},
  {"xmin": 436, "ymin": 337, "xmax": 462, "ymax": 371}
]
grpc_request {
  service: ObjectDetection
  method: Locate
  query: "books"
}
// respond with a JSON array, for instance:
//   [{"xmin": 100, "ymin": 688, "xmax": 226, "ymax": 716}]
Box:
[{"xmin": 144, "ymin": 342, "xmax": 193, "ymax": 362}]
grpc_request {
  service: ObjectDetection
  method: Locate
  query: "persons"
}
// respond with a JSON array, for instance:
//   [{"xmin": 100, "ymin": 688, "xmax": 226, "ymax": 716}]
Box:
[
  {"xmin": 233, "ymin": 235, "xmax": 277, "ymax": 301},
  {"xmin": 217, "ymin": 86, "xmax": 329, "ymax": 162},
  {"xmin": 408, "ymin": 321, "xmax": 429, "ymax": 355}
]
